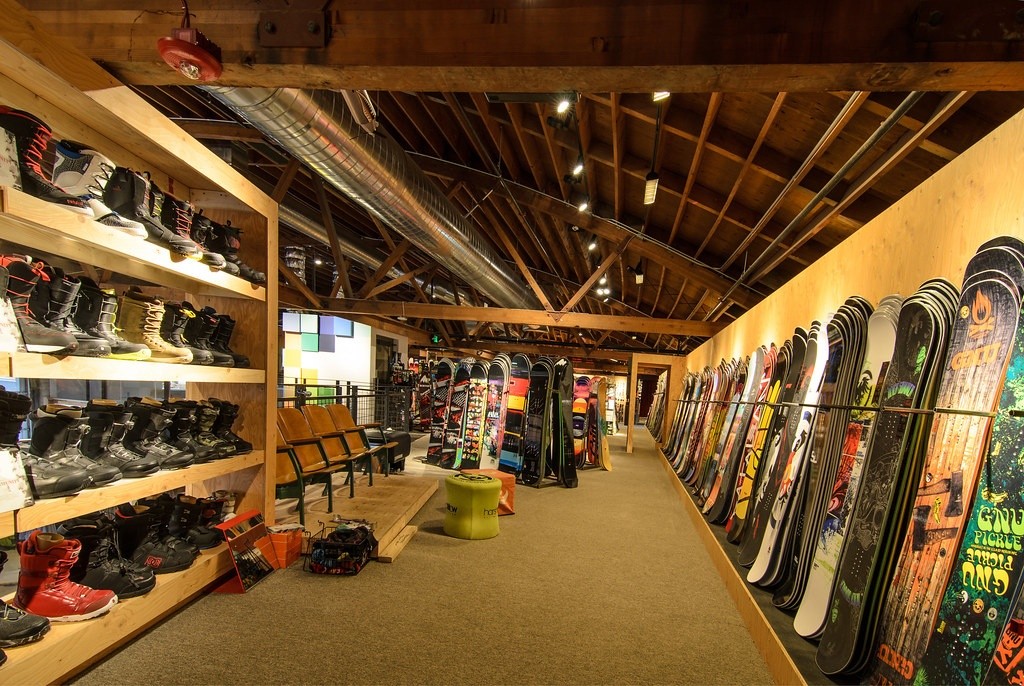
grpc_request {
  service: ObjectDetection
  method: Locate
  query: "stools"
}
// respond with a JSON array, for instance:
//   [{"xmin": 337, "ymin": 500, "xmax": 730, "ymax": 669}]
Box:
[{"xmin": 445, "ymin": 474, "xmax": 500, "ymax": 540}]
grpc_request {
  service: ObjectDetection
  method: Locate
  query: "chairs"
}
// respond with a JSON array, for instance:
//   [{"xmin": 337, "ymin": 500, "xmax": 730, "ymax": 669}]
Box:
[{"xmin": 275, "ymin": 404, "xmax": 399, "ymax": 528}]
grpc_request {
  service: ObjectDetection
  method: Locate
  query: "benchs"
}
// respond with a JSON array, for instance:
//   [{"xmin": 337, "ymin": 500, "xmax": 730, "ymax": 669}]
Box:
[{"xmin": 365, "ymin": 432, "xmax": 411, "ymax": 472}]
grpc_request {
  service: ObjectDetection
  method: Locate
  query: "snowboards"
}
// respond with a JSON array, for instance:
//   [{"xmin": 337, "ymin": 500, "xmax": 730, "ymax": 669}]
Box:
[
  {"xmin": 646, "ymin": 234, "xmax": 1024, "ymax": 686},
  {"xmin": 419, "ymin": 371, "xmax": 431, "ymax": 428},
  {"xmin": 572, "ymin": 376, "xmax": 613, "ymax": 472},
  {"xmin": 426, "ymin": 353, "xmax": 578, "ymax": 490}
]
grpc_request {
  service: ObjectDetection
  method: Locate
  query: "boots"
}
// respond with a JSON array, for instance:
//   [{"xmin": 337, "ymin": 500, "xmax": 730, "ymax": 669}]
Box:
[
  {"xmin": 0, "ymin": 105, "xmax": 267, "ymax": 366},
  {"xmin": 29, "ymin": 404, "xmax": 123, "ymax": 489},
  {"xmin": 164, "ymin": 400, "xmax": 218, "ymax": 462},
  {"xmin": 83, "ymin": 399, "xmax": 159, "ymax": 477},
  {"xmin": 0, "ymin": 385, "xmax": 35, "ymax": 514},
  {"xmin": 20, "ymin": 450, "xmax": 94, "ymax": 500},
  {"xmin": 124, "ymin": 396, "xmax": 195, "ymax": 468},
  {"xmin": 0, "ymin": 599, "xmax": 51, "ymax": 648},
  {"xmin": 135, "ymin": 494, "xmax": 200, "ymax": 558},
  {"xmin": 0, "ymin": 648, "xmax": 7, "ymax": 666},
  {"xmin": 56, "ymin": 513, "xmax": 156, "ymax": 598},
  {"xmin": 213, "ymin": 490, "xmax": 238, "ymax": 522},
  {"xmin": 12, "ymin": 530, "xmax": 118, "ymax": 622},
  {"xmin": 208, "ymin": 397, "xmax": 253, "ymax": 455},
  {"xmin": 177, "ymin": 494, "xmax": 222, "ymax": 548},
  {"xmin": 197, "ymin": 497, "xmax": 236, "ymax": 542},
  {"xmin": 191, "ymin": 399, "xmax": 237, "ymax": 458},
  {"xmin": 114, "ymin": 502, "xmax": 193, "ymax": 574}
]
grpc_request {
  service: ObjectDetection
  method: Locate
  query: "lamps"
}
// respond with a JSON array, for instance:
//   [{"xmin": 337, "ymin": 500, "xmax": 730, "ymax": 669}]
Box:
[
  {"xmin": 547, "ymin": 105, "xmax": 575, "ymax": 131},
  {"xmin": 563, "ymin": 172, "xmax": 583, "ymax": 185},
  {"xmin": 643, "ymin": 172, "xmax": 660, "ymax": 206},
  {"xmin": 635, "ymin": 269, "xmax": 644, "ymax": 286}
]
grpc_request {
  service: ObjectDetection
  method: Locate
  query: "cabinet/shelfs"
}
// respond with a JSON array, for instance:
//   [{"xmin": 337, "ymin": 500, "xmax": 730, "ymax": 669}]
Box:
[{"xmin": 0, "ymin": 0, "xmax": 280, "ymax": 686}]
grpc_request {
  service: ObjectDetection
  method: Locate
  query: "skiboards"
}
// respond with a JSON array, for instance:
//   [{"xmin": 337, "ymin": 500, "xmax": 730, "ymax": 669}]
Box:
[{"xmin": 408, "ymin": 358, "xmax": 438, "ymax": 424}]
